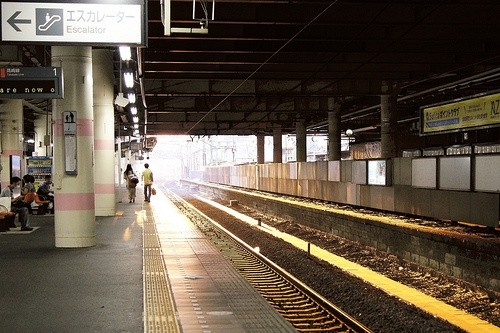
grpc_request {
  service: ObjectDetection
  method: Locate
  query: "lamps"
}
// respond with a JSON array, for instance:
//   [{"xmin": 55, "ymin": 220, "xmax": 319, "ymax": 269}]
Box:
[{"xmin": 120, "ymin": 46, "xmax": 141, "ymax": 143}]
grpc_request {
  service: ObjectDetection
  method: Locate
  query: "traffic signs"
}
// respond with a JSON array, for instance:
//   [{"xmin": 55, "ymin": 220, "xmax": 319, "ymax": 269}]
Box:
[{"xmin": 1, "ymin": 2, "xmax": 148, "ymax": 48}]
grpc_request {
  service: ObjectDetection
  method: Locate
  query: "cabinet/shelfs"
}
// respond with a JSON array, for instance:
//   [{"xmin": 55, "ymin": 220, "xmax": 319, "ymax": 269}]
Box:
[{"xmin": 25, "ymin": 157, "xmax": 54, "ymax": 194}]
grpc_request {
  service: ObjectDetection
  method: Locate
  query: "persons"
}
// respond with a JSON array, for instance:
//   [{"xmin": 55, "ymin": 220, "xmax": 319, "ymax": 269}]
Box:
[
  {"xmin": 1, "ymin": 174, "xmax": 54, "ymax": 232},
  {"xmin": 124, "ymin": 164, "xmax": 138, "ymax": 204},
  {"xmin": 141, "ymin": 163, "xmax": 154, "ymax": 202}
]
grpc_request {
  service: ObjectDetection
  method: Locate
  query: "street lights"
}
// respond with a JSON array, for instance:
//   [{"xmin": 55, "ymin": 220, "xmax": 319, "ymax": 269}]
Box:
[{"xmin": 346, "ymin": 129, "xmax": 353, "ymax": 156}]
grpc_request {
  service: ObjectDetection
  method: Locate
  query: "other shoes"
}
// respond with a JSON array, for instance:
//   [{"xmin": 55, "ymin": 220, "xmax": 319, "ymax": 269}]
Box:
[
  {"xmin": 145, "ymin": 197, "xmax": 148, "ymax": 201},
  {"xmin": 148, "ymin": 201, "xmax": 150, "ymax": 202},
  {"xmin": 20, "ymin": 226, "xmax": 33, "ymax": 231},
  {"xmin": 129, "ymin": 200, "xmax": 135, "ymax": 203}
]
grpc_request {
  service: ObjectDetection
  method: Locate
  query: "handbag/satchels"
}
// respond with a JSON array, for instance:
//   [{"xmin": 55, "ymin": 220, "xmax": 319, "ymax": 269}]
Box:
[
  {"xmin": 131, "ymin": 176, "xmax": 139, "ymax": 184},
  {"xmin": 151, "ymin": 187, "xmax": 156, "ymax": 196}
]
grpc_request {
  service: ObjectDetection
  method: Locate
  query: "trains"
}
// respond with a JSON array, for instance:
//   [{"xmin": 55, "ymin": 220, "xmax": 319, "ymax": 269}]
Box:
[{"xmin": 186, "ymin": 141, "xmax": 234, "ymax": 170}]
